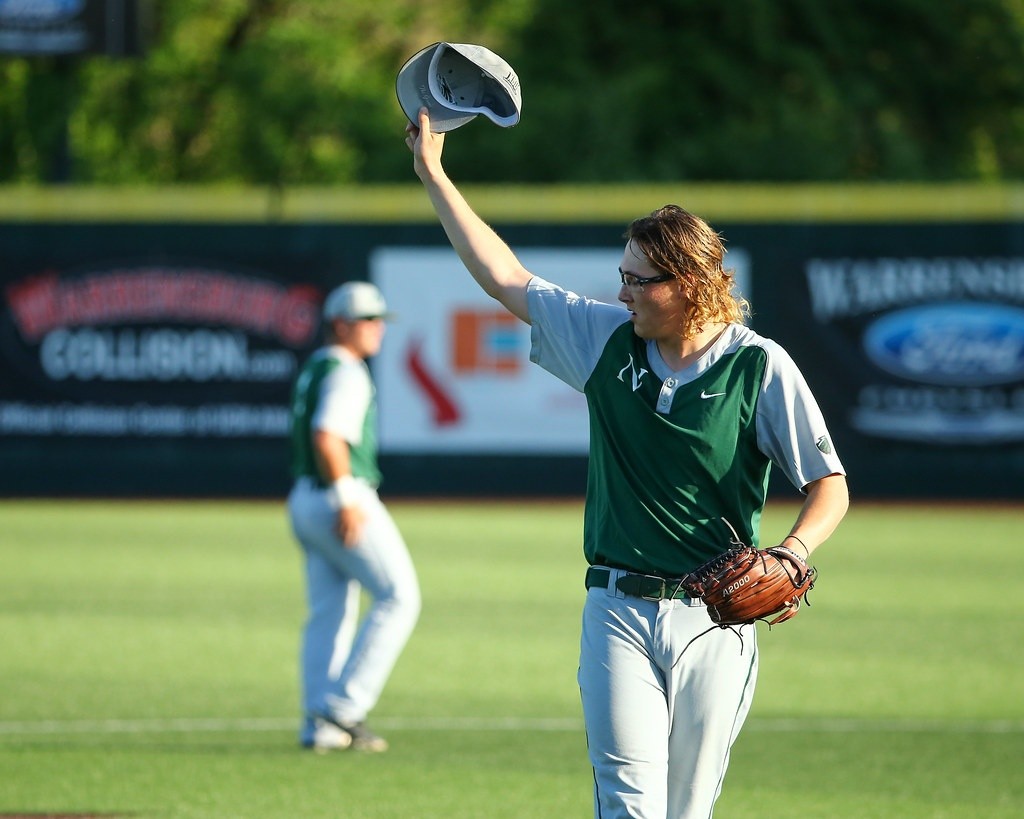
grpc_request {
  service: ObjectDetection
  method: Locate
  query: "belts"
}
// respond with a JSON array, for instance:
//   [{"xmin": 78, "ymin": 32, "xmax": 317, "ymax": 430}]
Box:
[{"xmin": 585, "ymin": 567, "xmax": 691, "ymax": 601}]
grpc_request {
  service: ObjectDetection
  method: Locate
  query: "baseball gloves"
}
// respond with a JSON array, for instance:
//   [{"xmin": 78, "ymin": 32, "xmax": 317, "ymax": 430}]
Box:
[{"xmin": 679, "ymin": 545, "xmax": 817, "ymax": 627}]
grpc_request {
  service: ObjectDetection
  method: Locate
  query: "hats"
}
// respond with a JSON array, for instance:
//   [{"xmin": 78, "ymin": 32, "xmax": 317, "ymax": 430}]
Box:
[
  {"xmin": 396, "ymin": 42, "xmax": 522, "ymax": 133},
  {"xmin": 325, "ymin": 280, "xmax": 398, "ymax": 321}
]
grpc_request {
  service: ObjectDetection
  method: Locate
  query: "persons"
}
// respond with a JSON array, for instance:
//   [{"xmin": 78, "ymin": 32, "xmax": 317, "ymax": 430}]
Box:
[
  {"xmin": 288, "ymin": 283, "xmax": 420, "ymax": 749},
  {"xmin": 400, "ymin": 106, "xmax": 848, "ymax": 819}
]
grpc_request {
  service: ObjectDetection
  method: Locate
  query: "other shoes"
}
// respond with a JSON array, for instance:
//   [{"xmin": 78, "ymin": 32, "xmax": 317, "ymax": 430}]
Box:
[{"xmin": 298, "ymin": 711, "xmax": 390, "ymax": 752}]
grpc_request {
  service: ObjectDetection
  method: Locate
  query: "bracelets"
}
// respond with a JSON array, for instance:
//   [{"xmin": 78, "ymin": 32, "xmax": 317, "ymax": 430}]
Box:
[
  {"xmin": 334, "ymin": 478, "xmax": 355, "ymax": 507},
  {"xmin": 786, "ymin": 536, "xmax": 809, "ymax": 554}
]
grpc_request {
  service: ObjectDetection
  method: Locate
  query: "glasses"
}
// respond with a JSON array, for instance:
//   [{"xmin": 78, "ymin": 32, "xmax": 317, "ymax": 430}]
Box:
[{"xmin": 619, "ymin": 267, "xmax": 678, "ymax": 293}]
